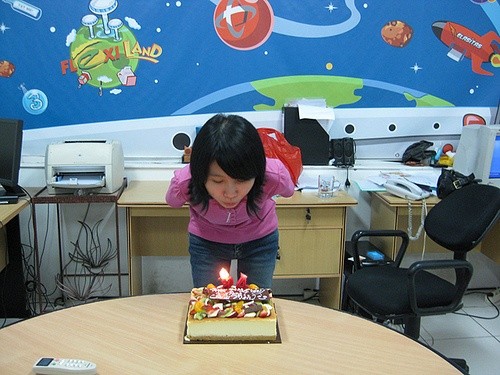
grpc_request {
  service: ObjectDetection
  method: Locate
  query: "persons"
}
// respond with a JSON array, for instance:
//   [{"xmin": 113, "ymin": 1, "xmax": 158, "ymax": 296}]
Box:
[{"xmin": 166, "ymin": 115, "xmax": 295, "ymax": 290}]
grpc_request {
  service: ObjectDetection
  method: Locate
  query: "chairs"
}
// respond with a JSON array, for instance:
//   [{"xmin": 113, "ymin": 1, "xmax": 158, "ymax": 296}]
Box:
[{"xmin": 343, "ymin": 184, "xmax": 500, "ymax": 342}]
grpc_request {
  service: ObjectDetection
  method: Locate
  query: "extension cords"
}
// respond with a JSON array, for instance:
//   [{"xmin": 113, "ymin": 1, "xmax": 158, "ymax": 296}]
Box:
[{"xmin": 484, "ymin": 288, "xmax": 500, "ymax": 306}]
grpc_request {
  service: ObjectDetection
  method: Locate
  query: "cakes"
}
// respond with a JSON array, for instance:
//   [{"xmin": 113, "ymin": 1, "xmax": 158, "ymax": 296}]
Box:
[{"xmin": 186, "ymin": 268, "xmax": 277, "ymax": 339}]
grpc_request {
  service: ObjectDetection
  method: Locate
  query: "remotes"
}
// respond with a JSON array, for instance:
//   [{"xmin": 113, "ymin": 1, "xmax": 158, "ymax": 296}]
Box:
[{"xmin": 32, "ymin": 356, "xmax": 95, "ymax": 375}]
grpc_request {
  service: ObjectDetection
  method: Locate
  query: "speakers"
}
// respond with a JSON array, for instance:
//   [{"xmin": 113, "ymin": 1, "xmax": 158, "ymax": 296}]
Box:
[
  {"xmin": 344, "ymin": 138, "xmax": 354, "ymax": 166},
  {"xmin": 333, "ymin": 139, "xmax": 345, "ymax": 166}
]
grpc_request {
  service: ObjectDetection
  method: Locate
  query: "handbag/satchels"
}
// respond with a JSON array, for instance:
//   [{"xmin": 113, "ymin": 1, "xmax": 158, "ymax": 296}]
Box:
[{"xmin": 436, "ymin": 167, "xmax": 482, "ymax": 199}]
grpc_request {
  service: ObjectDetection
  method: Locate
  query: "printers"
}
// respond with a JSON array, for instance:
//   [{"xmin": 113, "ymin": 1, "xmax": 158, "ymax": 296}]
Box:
[{"xmin": 44, "ymin": 137, "xmax": 125, "ymax": 195}]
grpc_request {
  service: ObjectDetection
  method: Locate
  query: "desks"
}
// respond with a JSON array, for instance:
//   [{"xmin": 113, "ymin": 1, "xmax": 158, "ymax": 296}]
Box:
[
  {"xmin": 117, "ymin": 180, "xmax": 362, "ymax": 312},
  {"xmin": 1, "ymin": 185, "xmax": 47, "ymax": 319},
  {"xmin": 369, "ymin": 191, "xmax": 500, "ymax": 282}
]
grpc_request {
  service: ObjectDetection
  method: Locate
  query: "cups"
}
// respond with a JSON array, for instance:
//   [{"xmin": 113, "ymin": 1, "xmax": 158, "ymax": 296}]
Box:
[{"xmin": 318, "ymin": 174, "xmax": 341, "ymax": 198}]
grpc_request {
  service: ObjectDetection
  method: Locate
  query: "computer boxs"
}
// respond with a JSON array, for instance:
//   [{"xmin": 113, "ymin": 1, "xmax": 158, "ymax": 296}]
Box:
[{"xmin": 344, "ymin": 240, "xmax": 393, "ymax": 280}]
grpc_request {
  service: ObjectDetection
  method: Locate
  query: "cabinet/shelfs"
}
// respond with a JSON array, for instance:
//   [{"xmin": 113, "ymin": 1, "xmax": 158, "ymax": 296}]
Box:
[{"xmin": 32, "ymin": 177, "xmax": 126, "ymax": 315}]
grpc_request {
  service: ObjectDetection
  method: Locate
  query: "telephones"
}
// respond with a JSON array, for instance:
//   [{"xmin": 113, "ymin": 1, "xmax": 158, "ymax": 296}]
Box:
[{"xmin": 384, "ymin": 174, "xmax": 430, "ymax": 241}]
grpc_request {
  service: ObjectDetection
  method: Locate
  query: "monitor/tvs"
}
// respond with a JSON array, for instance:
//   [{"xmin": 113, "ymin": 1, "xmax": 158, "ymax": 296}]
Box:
[
  {"xmin": 453, "ymin": 124, "xmax": 500, "ymax": 183},
  {"xmin": 0, "ymin": 117, "xmax": 24, "ymax": 187}
]
